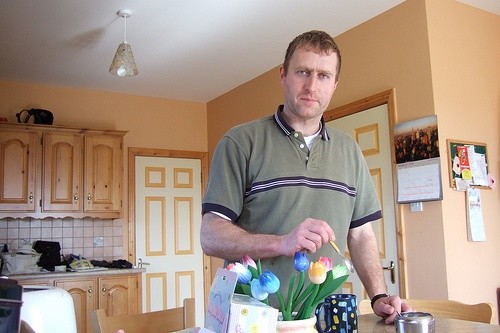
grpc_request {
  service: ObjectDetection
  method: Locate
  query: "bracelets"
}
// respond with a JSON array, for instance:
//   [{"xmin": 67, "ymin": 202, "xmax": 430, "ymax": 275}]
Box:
[{"xmin": 371, "ymin": 294, "xmax": 390, "ymax": 309}]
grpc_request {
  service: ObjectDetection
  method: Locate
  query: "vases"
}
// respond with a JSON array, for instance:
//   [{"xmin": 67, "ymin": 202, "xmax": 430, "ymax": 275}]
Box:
[{"xmin": 276, "ymin": 310, "xmax": 318, "ymax": 333}]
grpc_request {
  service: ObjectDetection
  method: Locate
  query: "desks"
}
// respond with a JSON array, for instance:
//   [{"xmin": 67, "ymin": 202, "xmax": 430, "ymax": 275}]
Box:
[{"xmin": 357, "ymin": 313, "xmax": 500, "ymax": 333}]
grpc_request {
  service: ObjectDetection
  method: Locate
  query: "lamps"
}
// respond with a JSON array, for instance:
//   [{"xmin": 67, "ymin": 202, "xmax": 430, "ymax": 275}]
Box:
[{"xmin": 108, "ymin": 9, "xmax": 139, "ymax": 77}]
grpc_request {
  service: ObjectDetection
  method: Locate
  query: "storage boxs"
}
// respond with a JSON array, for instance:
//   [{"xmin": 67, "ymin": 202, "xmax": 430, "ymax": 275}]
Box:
[{"xmin": 226, "ymin": 291, "xmax": 279, "ymax": 333}]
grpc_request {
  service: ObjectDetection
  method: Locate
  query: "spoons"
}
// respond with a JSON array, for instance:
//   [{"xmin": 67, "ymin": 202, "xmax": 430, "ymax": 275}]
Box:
[{"xmin": 329, "ymin": 241, "xmax": 355, "ymax": 274}]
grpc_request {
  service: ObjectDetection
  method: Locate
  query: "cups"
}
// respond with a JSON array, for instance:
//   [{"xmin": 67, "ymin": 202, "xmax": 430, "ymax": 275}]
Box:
[
  {"xmin": 394, "ymin": 312, "xmax": 435, "ymax": 333},
  {"xmin": 315, "ymin": 294, "xmax": 359, "ymax": 333}
]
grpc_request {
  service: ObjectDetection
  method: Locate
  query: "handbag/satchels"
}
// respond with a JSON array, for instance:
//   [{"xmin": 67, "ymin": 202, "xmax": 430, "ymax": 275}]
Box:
[
  {"xmin": 1, "ymin": 239, "xmax": 41, "ymax": 275},
  {"xmin": 35, "ymin": 241, "xmax": 61, "ymax": 271}
]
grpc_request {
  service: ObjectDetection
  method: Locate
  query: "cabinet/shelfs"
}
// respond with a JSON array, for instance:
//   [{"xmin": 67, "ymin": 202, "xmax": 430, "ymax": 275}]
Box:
[
  {"xmin": 18, "ymin": 271, "xmax": 143, "ymax": 333},
  {"xmin": 0, "ymin": 121, "xmax": 129, "ymax": 220}
]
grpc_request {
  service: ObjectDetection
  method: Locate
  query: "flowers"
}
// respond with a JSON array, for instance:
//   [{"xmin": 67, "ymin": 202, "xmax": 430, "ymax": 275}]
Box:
[{"xmin": 225, "ymin": 248, "xmax": 351, "ymax": 321}]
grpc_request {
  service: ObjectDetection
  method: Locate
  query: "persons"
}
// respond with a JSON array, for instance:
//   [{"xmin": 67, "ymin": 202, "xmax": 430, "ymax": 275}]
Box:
[{"xmin": 199, "ymin": 29, "xmax": 416, "ymax": 325}]
